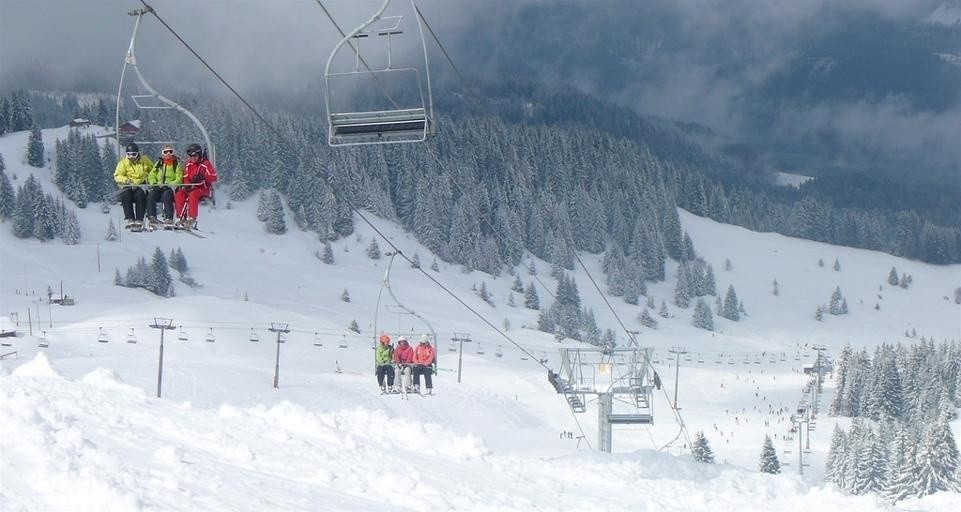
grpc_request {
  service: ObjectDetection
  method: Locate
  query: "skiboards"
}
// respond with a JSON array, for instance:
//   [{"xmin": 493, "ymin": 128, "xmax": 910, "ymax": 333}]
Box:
[
  {"xmin": 128, "ymin": 222, "xmax": 204, "ymax": 237},
  {"xmin": 381, "ymin": 387, "xmax": 436, "ymax": 398}
]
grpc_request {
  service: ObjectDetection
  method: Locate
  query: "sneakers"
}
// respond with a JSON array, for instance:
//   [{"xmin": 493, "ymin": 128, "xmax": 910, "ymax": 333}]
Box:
[
  {"xmin": 381, "ymin": 385, "xmax": 433, "ymax": 394},
  {"xmin": 124, "ymin": 216, "xmax": 198, "ymax": 230}
]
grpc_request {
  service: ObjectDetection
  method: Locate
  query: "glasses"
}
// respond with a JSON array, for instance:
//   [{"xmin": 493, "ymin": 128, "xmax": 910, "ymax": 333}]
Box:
[
  {"xmin": 126, "ymin": 152, "xmax": 139, "ymax": 158},
  {"xmin": 162, "ymin": 149, "xmax": 174, "ymax": 156},
  {"xmin": 187, "ymin": 151, "xmax": 200, "ymax": 156}
]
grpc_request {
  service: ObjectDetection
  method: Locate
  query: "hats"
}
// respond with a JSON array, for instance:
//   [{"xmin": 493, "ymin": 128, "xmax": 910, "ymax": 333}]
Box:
[
  {"xmin": 380, "ymin": 336, "xmax": 390, "ymax": 342},
  {"xmin": 398, "ymin": 336, "xmax": 407, "ymax": 343},
  {"xmin": 420, "ymin": 335, "xmax": 429, "ymax": 344}
]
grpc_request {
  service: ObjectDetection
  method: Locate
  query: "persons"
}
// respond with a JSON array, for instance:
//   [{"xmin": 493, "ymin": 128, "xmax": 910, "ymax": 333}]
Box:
[
  {"xmin": 375, "ymin": 335, "xmax": 438, "ymax": 394},
  {"xmin": 113, "ymin": 142, "xmax": 218, "ymax": 230}
]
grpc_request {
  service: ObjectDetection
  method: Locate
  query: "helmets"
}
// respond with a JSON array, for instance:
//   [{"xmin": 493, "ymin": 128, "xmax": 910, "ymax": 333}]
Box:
[
  {"xmin": 126, "ymin": 144, "xmax": 138, "ymax": 152},
  {"xmin": 187, "ymin": 144, "xmax": 201, "ymax": 155}
]
